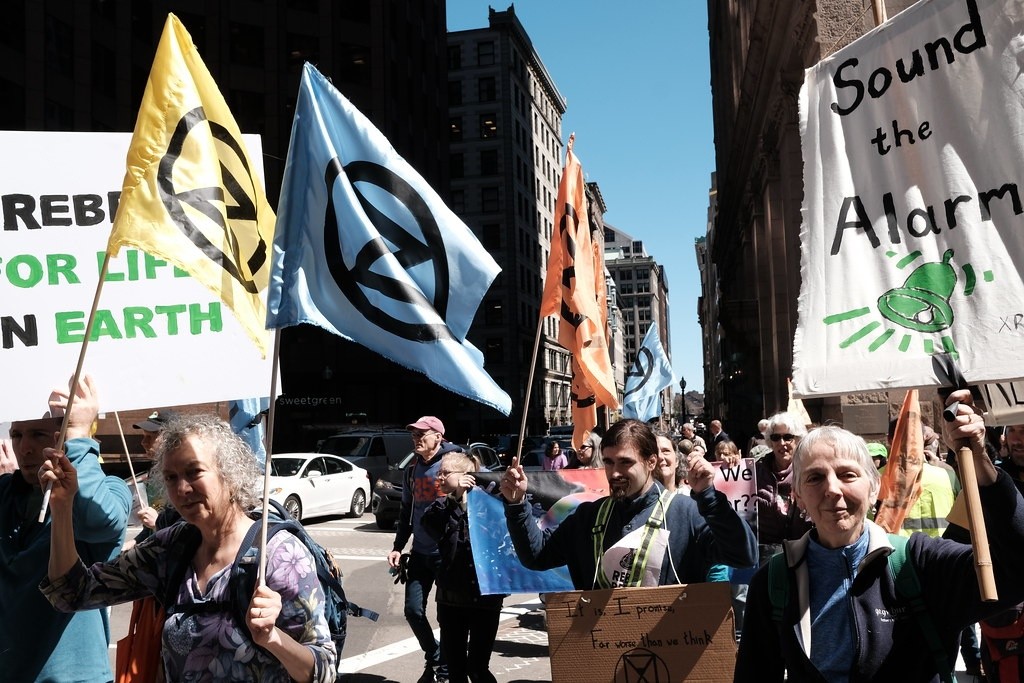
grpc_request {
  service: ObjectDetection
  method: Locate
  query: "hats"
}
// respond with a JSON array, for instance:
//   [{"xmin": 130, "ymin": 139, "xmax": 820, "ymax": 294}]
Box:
[
  {"xmin": 865, "ymin": 443, "xmax": 887, "ymax": 459},
  {"xmin": 406, "ymin": 416, "xmax": 445, "ymax": 435}
]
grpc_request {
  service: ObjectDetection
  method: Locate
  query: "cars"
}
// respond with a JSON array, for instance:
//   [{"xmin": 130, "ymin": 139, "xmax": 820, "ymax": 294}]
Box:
[
  {"xmin": 469, "ymin": 427, "xmax": 580, "ymax": 469},
  {"xmin": 257, "ymin": 454, "xmax": 373, "ymax": 521}
]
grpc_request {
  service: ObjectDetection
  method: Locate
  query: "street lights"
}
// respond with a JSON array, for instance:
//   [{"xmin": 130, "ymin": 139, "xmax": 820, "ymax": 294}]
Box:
[{"xmin": 679, "ymin": 376, "xmax": 687, "ymax": 423}]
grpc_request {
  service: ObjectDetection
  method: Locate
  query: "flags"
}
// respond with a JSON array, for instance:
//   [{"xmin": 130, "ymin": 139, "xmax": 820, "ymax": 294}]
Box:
[
  {"xmin": 265, "ymin": 62, "xmax": 512, "ymax": 416},
  {"xmin": 624, "ymin": 324, "xmax": 677, "ymax": 422},
  {"xmin": 542, "ymin": 148, "xmax": 619, "ymax": 451},
  {"xmin": 874, "ymin": 389, "xmax": 923, "ymax": 534},
  {"xmin": 109, "ymin": 14, "xmax": 277, "ymax": 360}
]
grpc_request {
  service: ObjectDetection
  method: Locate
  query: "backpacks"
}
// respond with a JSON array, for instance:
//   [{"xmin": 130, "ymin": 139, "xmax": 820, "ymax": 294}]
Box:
[{"xmin": 159, "ymin": 496, "xmax": 349, "ymax": 675}]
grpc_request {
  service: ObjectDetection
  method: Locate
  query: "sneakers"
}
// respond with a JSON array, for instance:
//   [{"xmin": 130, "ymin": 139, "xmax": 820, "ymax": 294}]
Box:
[{"xmin": 418, "ymin": 663, "xmax": 439, "ymax": 683}]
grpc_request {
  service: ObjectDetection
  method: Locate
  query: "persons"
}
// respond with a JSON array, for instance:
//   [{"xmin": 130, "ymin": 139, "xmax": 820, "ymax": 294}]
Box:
[
  {"xmin": 387, "ymin": 415, "xmax": 505, "ymax": 683},
  {"xmin": 0, "ymin": 372, "xmax": 133, "ymax": 683},
  {"xmin": 499, "ymin": 419, "xmax": 760, "ymax": 590},
  {"xmin": 735, "ymin": 390, "xmax": 1024, "ymax": 683},
  {"xmin": 133, "ymin": 409, "xmax": 182, "ymax": 544},
  {"xmin": 542, "ymin": 411, "xmax": 808, "ymax": 522},
  {"xmin": 37, "ymin": 413, "xmax": 337, "ymax": 683}
]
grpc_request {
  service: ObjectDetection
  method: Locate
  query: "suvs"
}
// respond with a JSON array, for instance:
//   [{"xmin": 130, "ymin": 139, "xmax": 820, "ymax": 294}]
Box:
[
  {"xmin": 375, "ymin": 443, "xmax": 505, "ymax": 528},
  {"xmin": 319, "ymin": 431, "xmax": 418, "ymax": 482}
]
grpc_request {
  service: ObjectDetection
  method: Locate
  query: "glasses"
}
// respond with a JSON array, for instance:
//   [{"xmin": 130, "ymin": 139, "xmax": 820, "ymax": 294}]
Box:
[
  {"xmin": 437, "ymin": 469, "xmax": 465, "ymax": 477},
  {"xmin": 770, "ymin": 433, "xmax": 794, "ymax": 441},
  {"xmin": 580, "ymin": 445, "xmax": 595, "ymax": 448},
  {"xmin": 411, "ymin": 431, "xmax": 439, "ymax": 437}
]
